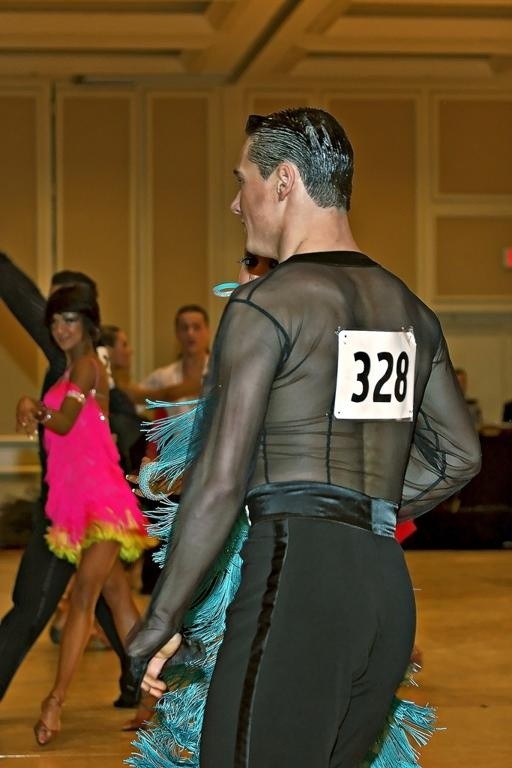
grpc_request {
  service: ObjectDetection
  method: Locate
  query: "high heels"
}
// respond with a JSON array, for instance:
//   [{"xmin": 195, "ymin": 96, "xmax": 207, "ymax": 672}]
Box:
[
  {"xmin": 36, "ymin": 695, "xmax": 65, "ymax": 746},
  {"xmin": 121, "ymin": 689, "xmax": 168, "ymax": 731}
]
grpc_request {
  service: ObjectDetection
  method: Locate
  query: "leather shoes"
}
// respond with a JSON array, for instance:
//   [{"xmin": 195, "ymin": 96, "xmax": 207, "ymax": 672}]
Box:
[{"xmin": 114, "ymin": 676, "xmax": 138, "ymax": 708}]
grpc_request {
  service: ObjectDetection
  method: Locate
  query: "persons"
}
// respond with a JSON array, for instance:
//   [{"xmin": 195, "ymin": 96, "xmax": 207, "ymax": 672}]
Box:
[
  {"xmin": 454, "ymin": 365, "xmax": 484, "ymax": 431},
  {"xmin": 0, "ymin": 249, "xmax": 278, "ymax": 747},
  {"xmin": 122, "ymin": 102, "xmax": 486, "ymax": 768}
]
